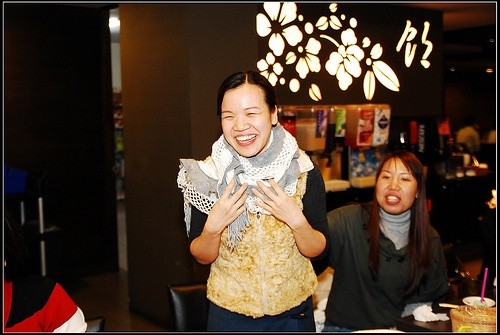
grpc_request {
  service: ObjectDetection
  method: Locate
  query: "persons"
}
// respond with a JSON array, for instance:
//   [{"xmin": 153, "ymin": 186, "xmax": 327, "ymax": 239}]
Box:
[
  {"xmin": 309, "ymin": 149, "xmax": 449, "ymax": 335},
  {"xmin": 177, "ymin": 71, "xmax": 329, "ymax": 335},
  {"xmin": 456, "ymin": 118, "xmax": 485, "ymax": 150},
  {"xmin": 0, "ymin": 203, "xmax": 88, "ymax": 335}
]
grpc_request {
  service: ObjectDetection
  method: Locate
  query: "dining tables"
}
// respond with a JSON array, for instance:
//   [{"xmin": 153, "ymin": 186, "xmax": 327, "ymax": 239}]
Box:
[{"xmin": 396, "ymin": 299, "xmax": 497, "ymax": 335}]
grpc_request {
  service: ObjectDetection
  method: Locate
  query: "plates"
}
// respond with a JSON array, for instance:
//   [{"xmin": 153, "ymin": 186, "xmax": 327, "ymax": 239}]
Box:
[{"xmin": 463, "ymin": 297, "xmax": 495, "ymax": 307}]
